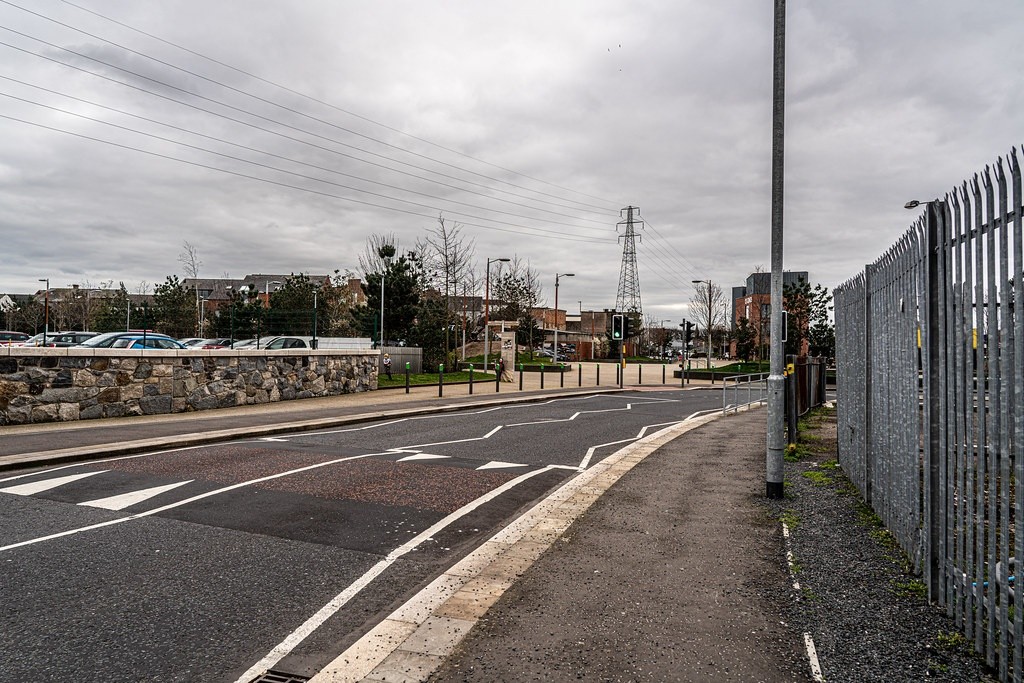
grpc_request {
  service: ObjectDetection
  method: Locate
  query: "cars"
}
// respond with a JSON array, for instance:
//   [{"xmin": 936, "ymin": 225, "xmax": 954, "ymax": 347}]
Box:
[{"xmin": 0, "ymin": 329, "xmax": 319, "ymax": 350}]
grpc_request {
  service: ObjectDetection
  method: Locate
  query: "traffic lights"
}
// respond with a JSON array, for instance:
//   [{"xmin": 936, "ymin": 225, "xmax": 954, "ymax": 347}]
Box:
[
  {"xmin": 686, "ymin": 321, "xmax": 695, "ymax": 343},
  {"xmin": 612, "ymin": 315, "xmax": 623, "ymax": 340}
]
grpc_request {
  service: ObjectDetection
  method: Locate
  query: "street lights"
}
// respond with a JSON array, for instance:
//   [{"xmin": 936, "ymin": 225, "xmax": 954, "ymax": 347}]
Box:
[
  {"xmin": 39, "ymin": 279, "xmax": 49, "ymax": 346},
  {"xmin": 87, "ymin": 290, "xmax": 103, "ymax": 332},
  {"xmin": 265, "ymin": 281, "xmax": 281, "ymax": 308},
  {"xmin": 125, "ymin": 299, "xmax": 129, "ymax": 332},
  {"xmin": 720, "ymin": 303, "xmax": 727, "ymax": 354},
  {"xmin": 200, "ymin": 296, "xmax": 203, "ymax": 338},
  {"xmin": 449, "ymin": 281, "xmax": 466, "ymax": 362},
  {"xmin": 312, "ymin": 291, "xmax": 317, "ymax": 350},
  {"xmin": 484, "ymin": 258, "xmax": 510, "ymax": 377},
  {"xmin": 692, "ymin": 280, "xmax": 712, "ymax": 371},
  {"xmin": 553, "ymin": 273, "xmax": 575, "ymax": 364}
]
